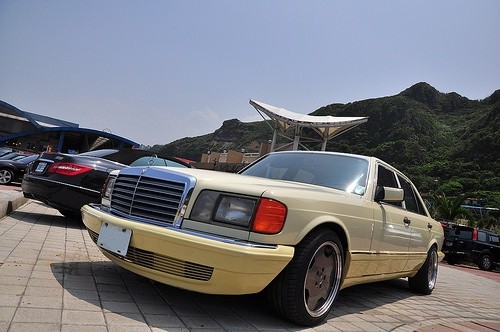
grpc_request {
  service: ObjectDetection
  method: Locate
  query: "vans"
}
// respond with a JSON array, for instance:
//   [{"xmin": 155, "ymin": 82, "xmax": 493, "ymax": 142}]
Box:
[{"xmin": 440, "ymin": 221, "xmax": 500, "ymax": 272}]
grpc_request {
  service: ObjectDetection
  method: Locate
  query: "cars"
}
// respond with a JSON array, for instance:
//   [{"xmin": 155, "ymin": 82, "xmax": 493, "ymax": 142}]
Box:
[
  {"xmin": 22, "ymin": 148, "xmax": 204, "ymax": 228},
  {"xmin": 80, "ymin": 149, "xmax": 445, "ymax": 328},
  {"xmin": 0, "ymin": 146, "xmax": 41, "ymax": 185}
]
